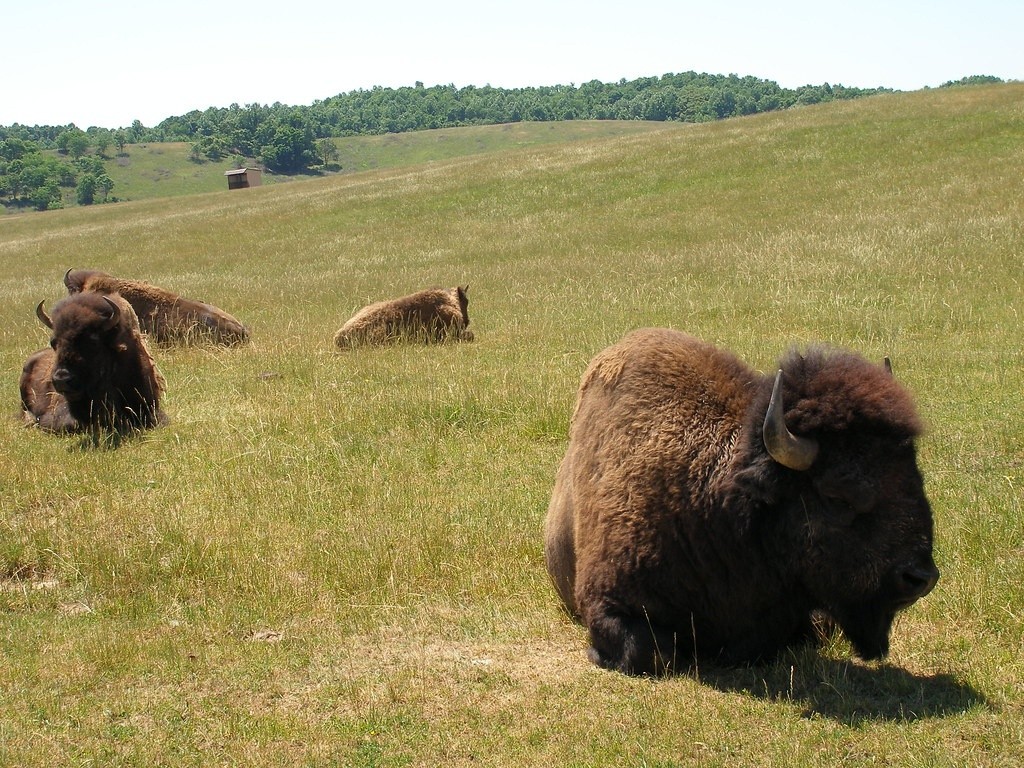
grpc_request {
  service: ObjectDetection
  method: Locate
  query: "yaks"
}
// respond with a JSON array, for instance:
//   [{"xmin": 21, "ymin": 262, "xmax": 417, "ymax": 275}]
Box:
[
  {"xmin": 333, "ymin": 282, "xmax": 476, "ymax": 352},
  {"xmin": 18, "ymin": 265, "xmax": 254, "ymax": 456},
  {"xmin": 544, "ymin": 328, "xmax": 939, "ymax": 681}
]
